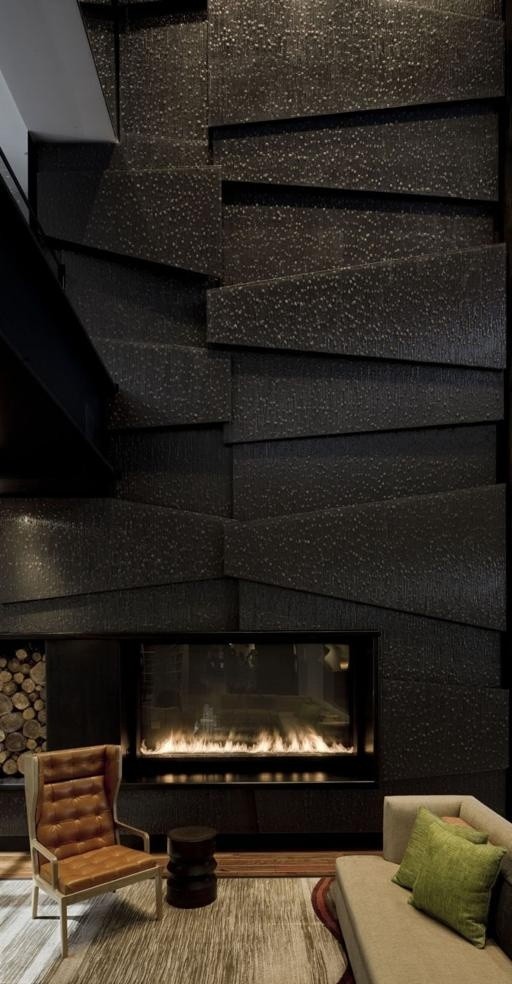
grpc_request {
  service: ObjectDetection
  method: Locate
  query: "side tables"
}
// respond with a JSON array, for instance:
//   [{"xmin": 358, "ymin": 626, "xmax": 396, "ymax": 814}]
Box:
[{"xmin": 165, "ymin": 825, "xmax": 220, "ymax": 911}]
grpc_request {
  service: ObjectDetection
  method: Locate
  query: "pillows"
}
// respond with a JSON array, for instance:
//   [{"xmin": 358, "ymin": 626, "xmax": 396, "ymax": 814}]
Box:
[{"xmin": 387, "ymin": 799, "xmax": 511, "ymax": 960}]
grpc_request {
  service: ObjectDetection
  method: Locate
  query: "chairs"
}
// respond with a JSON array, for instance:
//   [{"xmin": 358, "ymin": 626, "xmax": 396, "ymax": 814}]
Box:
[{"xmin": 22, "ymin": 742, "xmax": 168, "ymax": 960}]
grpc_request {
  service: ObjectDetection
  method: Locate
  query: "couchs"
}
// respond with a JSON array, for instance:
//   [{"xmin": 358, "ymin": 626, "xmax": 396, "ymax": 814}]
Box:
[{"xmin": 331, "ymin": 786, "xmax": 509, "ymax": 984}]
